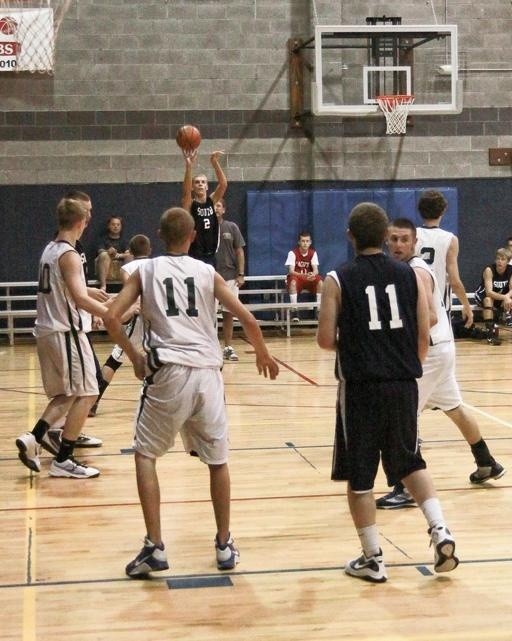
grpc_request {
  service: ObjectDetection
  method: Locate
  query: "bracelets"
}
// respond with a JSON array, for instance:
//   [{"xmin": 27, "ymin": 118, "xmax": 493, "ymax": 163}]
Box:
[{"xmin": 236, "ymin": 273, "xmax": 244, "ymax": 277}]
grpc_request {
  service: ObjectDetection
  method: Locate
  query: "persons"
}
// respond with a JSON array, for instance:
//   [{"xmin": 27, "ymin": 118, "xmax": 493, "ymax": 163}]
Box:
[
  {"xmin": 284, "ymin": 232, "xmax": 323, "ymax": 322},
  {"xmin": 16, "ymin": 192, "xmax": 151, "ymax": 479},
  {"xmin": 375, "ymin": 219, "xmax": 506, "ymax": 510},
  {"xmin": 503, "ymin": 237, "xmax": 512, "ymax": 326},
  {"xmin": 182, "ymin": 146, "xmax": 227, "ymax": 269},
  {"xmin": 214, "ymin": 198, "xmax": 246, "ymax": 360},
  {"xmin": 317, "ymin": 202, "xmax": 459, "ymax": 583},
  {"xmin": 415, "ymin": 190, "xmax": 474, "ymax": 330},
  {"xmin": 105, "ymin": 206, "xmax": 280, "ymax": 576},
  {"xmin": 474, "ymin": 248, "xmax": 512, "ymax": 344}
]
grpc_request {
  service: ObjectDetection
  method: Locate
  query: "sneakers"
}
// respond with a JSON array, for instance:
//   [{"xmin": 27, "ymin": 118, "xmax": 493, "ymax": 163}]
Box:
[
  {"xmin": 125, "ymin": 535, "xmax": 169, "ymax": 576},
  {"xmin": 291, "ymin": 308, "xmax": 301, "ymax": 322},
  {"xmin": 214, "ymin": 529, "xmax": 241, "ymax": 570},
  {"xmin": 75, "ymin": 430, "xmax": 103, "ymax": 448},
  {"xmin": 427, "ymin": 523, "xmax": 459, "ymax": 573},
  {"xmin": 505, "ymin": 316, "xmax": 512, "ymax": 327},
  {"xmin": 16, "ymin": 431, "xmax": 42, "ymax": 473},
  {"xmin": 40, "ymin": 425, "xmax": 64, "ymax": 457},
  {"xmin": 470, "ymin": 456, "xmax": 506, "ymax": 484},
  {"xmin": 486, "ymin": 328, "xmax": 501, "ymax": 347},
  {"xmin": 47, "ymin": 454, "xmax": 101, "ymax": 479},
  {"xmin": 374, "ymin": 481, "xmax": 416, "ymax": 509},
  {"xmin": 222, "ymin": 345, "xmax": 239, "ymax": 361},
  {"xmin": 345, "ymin": 546, "xmax": 389, "ymax": 583},
  {"xmin": 87, "ymin": 401, "xmax": 98, "ymax": 417}
]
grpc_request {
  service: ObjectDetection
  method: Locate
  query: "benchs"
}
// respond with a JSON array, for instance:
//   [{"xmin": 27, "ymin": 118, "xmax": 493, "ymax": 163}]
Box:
[
  {"xmin": 452, "ymin": 292, "xmax": 484, "ymax": 320},
  {"xmin": 1, "ymin": 279, "xmax": 140, "ymax": 347},
  {"xmin": 214, "ymin": 275, "xmax": 320, "ymax": 340}
]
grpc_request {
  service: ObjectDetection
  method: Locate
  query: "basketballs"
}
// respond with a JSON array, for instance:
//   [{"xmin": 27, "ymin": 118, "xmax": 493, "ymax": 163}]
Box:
[{"xmin": 176, "ymin": 126, "xmax": 200, "ymax": 150}]
